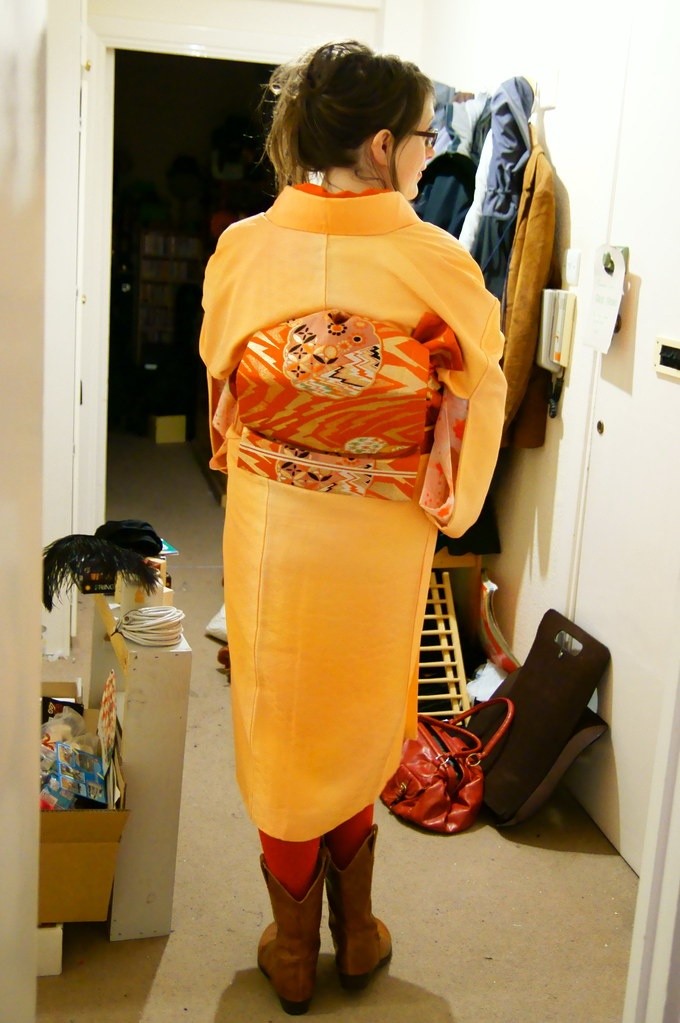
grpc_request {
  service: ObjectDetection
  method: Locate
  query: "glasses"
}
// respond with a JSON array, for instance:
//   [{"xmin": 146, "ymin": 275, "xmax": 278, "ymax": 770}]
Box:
[{"xmin": 386, "ymin": 126, "xmax": 438, "ymax": 147}]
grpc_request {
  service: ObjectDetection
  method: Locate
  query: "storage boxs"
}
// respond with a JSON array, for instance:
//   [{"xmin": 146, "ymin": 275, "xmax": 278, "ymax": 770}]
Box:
[
  {"xmin": 148, "ymin": 410, "xmax": 186, "ymax": 445},
  {"xmin": 32, "ymin": 679, "xmax": 132, "ymax": 982}
]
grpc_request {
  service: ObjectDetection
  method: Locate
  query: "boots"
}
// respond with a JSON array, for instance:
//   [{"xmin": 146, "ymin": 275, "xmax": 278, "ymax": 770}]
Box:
[
  {"xmin": 258, "ymin": 848, "xmax": 328, "ymax": 1017},
  {"xmin": 329, "ymin": 824, "xmax": 392, "ymax": 992}
]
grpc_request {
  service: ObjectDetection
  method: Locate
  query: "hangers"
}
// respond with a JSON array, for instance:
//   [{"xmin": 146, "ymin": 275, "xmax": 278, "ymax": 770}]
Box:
[{"xmin": 397, "ymin": 77, "xmax": 552, "ymax": 186}]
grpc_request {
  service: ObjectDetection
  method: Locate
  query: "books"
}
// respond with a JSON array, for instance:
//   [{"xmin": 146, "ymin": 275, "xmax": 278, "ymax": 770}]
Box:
[{"xmin": 142, "ymin": 233, "xmax": 203, "ymax": 344}]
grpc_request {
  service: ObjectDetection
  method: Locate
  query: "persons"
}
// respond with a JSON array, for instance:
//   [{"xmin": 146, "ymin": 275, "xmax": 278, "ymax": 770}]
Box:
[{"xmin": 200, "ymin": 40, "xmax": 509, "ymax": 1015}]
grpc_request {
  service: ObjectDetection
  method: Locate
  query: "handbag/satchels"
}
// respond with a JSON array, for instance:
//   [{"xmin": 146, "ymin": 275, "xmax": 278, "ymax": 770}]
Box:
[{"xmin": 380, "ymin": 696, "xmax": 514, "ymax": 834}]
